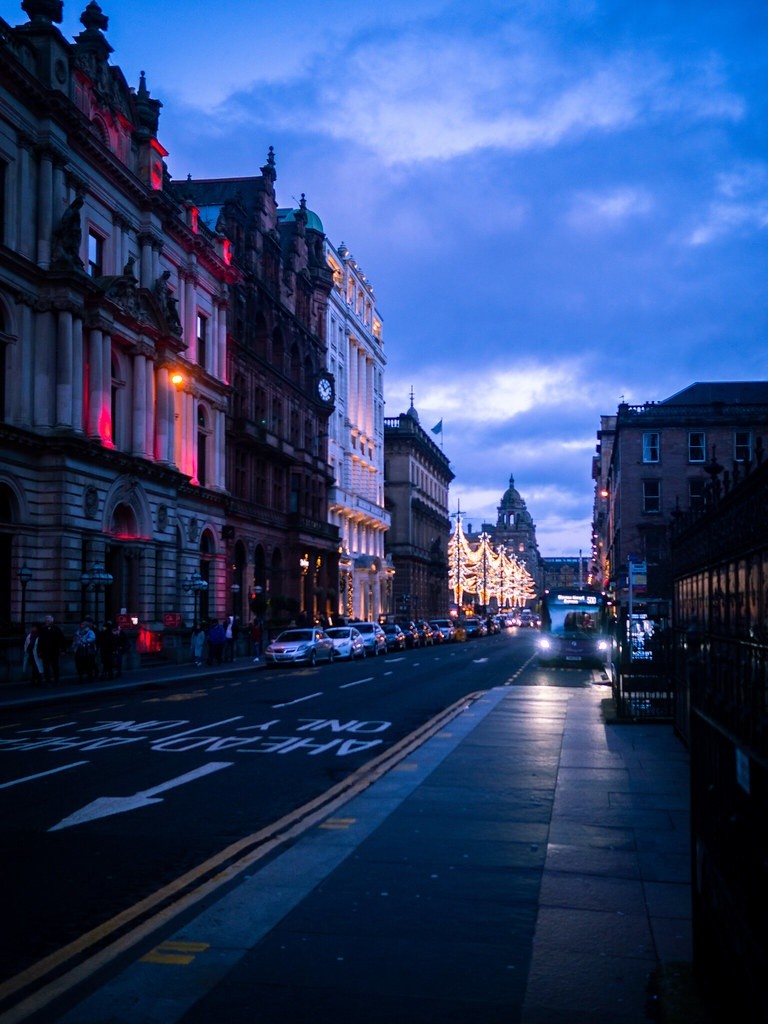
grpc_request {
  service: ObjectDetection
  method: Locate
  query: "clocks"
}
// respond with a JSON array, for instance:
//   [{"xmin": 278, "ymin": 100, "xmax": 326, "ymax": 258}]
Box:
[{"xmin": 318, "ymin": 378, "xmax": 332, "ymax": 402}]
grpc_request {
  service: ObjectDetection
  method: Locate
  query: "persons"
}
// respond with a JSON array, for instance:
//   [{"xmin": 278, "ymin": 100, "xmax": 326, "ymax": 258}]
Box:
[
  {"xmin": 190, "ymin": 616, "xmax": 237, "ymax": 666},
  {"xmin": 73, "ymin": 615, "xmax": 131, "ymax": 681},
  {"xmin": 23, "ymin": 615, "xmax": 62, "ymax": 687},
  {"xmin": 296, "ymin": 610, "xmax": 367, "ymax": 629},
  {"xmin": 583, "ymin": 614, "xmax": 595, "ymax": 632}
]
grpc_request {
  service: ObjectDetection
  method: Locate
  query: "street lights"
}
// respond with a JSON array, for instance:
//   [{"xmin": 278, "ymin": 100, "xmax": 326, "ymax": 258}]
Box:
[
  {"xmin": 182, "ymin": 570, "xmax": 209, "ymax": 632},
  {"xmin": 18, "ymin": 563, "xmax": 32, "ymax": 636},
  {"xmin": 80, "ymin": 561, "xmax": 113, "ymax": 629}
]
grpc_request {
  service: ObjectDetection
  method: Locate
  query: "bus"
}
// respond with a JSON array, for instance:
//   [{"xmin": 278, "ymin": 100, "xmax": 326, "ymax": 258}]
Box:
[{"xmin": 539, "ymin": 587, "xmax": 612, "ymax": 667}]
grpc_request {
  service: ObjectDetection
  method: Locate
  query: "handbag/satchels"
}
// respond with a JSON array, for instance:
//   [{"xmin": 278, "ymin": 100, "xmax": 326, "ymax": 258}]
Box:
[
  {"xmin": 80, "ymin": 630, "xmax": 92, "ymax": 648},
  {"xmin": 117, "ymin": 630, "xmax": 132, "ymax": 655}
]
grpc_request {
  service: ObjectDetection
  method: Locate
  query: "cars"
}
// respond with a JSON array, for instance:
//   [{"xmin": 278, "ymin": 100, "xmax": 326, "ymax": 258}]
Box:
[
  {"xmin": 326, "ymin": 627, "xmax": 367, "ymax": 660},
  {"xmin": 416, "ymin": 621, "xmax": 434, "ymax": 647},
  {"xmin": 481, "ymin": 609, "xmax": 541, "ymax": 636},
  {"xmin": 348, "ymin": 622, "xmax": 387, "ymax": 656},
  {"xmin": 381, "ymin": 624, "xmax": 406, "ymax": 651},
  {"xmin": 434, "ymin": 619, "xmax": 455, "ymax": 642},
  {"xmin": 399, "ymin": 621, "xmax": 420, "ymax": 649},
  {"xmin": 464, "ymin": 618, "xmax": 481, "ymax": 637},
  {"xmin": 430, "ymin": 623, "xmax": 444, "ymax": 645},
  {"xmin": 264, "ymin": 628, "xmax": 334, "ymax": 666}
]
